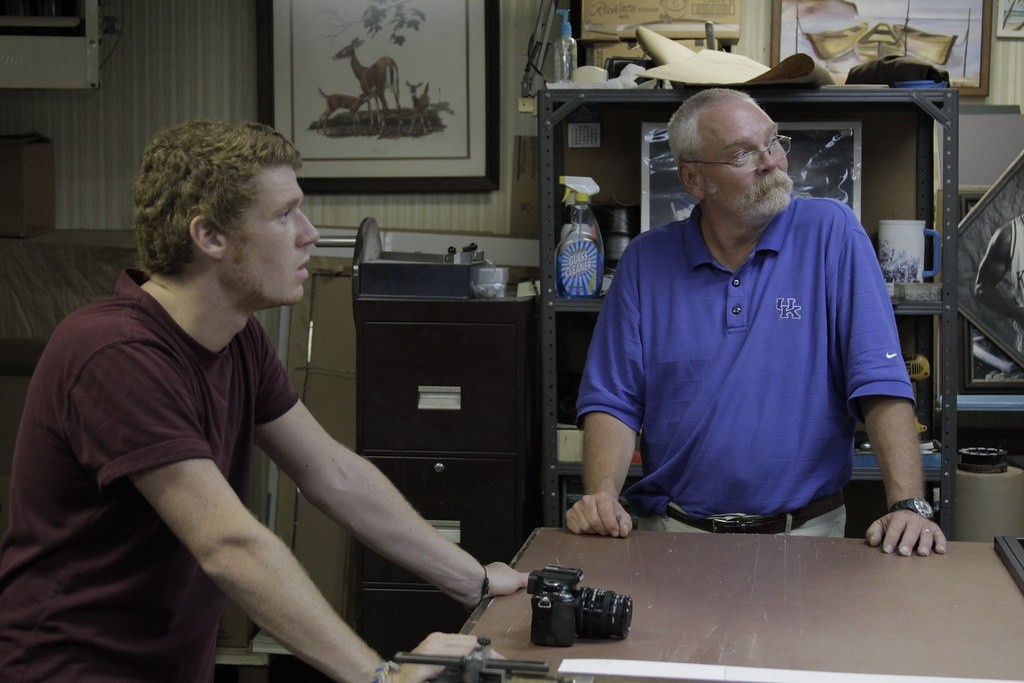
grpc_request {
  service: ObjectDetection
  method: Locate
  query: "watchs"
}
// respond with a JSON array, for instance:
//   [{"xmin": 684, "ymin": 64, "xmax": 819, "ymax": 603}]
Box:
[{"xmin": 890, "ymin": 496, "xmax": 934, "ymax": 518}]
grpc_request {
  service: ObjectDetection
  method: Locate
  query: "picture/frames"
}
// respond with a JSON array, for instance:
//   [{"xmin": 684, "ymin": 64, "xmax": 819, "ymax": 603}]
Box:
[
  {"xmin": 639, "ymin": 121, "xmax": 862, "ymax": 233},
  {"xmin": 253, "ymin": 0, "xmax": 502, "ymax": 196},
  {"xmin": 958, "ymin": 183, "xmax": 1024, "ymax": 396},
  {"xmin": 769, "ymin": 0, "xmax": 993, "ymax": 97},
  {"xmin": 958, "ymin": 149, "xmax": 1024, "ymax": 368}
]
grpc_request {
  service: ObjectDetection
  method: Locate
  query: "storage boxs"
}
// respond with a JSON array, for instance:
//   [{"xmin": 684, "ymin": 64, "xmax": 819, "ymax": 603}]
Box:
[
  {"xmin": 0, "ymin": 129, "xmax": 55, "ymax": 238},
  {"xmin": 556, "ymin": 421, "xmax": 585, "ymax": 462},
  {"xmin": 586, "ymin": 40, "xmax": 719, "ymax": 68},
  {"xmin": 574, "ymin": 0, "xmax": 741, "ymax": 46}
]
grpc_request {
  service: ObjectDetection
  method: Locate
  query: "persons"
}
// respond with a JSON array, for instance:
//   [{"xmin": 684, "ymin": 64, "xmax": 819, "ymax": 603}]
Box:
[
  {"xmin": 565, "ymin": 87, "xmax": 948, "ymax": 558},
  {"xmin": 0, "ymin": 120, "xmax": 529, "ymax": 683}
]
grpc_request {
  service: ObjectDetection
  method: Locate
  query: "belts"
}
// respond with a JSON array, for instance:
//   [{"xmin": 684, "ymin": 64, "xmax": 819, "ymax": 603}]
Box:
[{"xmin": 666, "ymin": 490, "xmax": 844, "ymax": 533}]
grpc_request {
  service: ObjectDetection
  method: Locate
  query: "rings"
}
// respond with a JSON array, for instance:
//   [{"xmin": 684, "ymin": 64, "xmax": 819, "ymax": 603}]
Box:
[{"xmin": 921, "ymin": 528, "xmax": 931, "ymax": 532}]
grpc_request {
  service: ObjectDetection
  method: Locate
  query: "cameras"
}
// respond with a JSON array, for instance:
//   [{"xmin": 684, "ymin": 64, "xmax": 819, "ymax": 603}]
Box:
[{"xmin": 527, "ymin": 565, "xmax": 632, "ymax": 647}]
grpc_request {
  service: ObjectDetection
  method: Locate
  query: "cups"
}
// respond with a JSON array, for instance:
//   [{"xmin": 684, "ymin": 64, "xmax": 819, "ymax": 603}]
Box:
[{"xmin": 878, "ymin": 220, "xmax": 940, "ymax": 295}]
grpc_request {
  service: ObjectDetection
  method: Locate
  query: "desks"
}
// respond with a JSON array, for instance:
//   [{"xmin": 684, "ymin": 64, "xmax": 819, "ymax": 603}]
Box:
[{"xmin": 459, "ymin": 528, "xmax": 1024, "ymax": 680}]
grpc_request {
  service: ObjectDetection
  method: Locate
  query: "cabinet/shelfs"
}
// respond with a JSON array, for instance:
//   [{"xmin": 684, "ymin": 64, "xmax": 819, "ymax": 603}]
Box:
[
  {"xmin": 537, "ymin": 88, "xmax": 961, "ymax": 540},
  {"xmin": 353, "ymin": 216, "xmax": 543, "ymax": 660}
]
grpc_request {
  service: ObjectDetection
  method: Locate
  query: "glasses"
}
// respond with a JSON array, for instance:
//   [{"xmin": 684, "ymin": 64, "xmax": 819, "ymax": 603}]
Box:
[{"xmin": 685, "ymin": 134, "xmax": 791, "ymax": 172}]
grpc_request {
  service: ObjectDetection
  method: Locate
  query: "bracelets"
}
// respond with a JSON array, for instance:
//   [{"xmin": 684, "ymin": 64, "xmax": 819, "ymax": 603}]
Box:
[
  {"xmin": 475, "ymin": 567, "xmax": 490, "ymax": 610},
  {"xmin": 371, "ymin": 657, "xmax": 401, "ymax": 683}
]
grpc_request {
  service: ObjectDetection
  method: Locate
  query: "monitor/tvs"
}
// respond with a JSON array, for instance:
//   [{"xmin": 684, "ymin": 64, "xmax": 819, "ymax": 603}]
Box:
[{"xmin": 641, "ymin": 121, "xmax": 861, "ymax": 227}]
